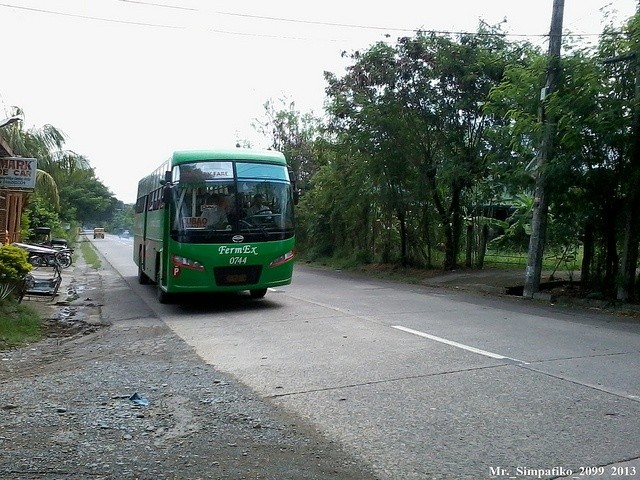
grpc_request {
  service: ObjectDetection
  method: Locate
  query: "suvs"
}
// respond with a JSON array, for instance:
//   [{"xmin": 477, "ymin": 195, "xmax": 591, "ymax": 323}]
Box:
[{"xmin": 94, "ymin": 228, "xmax": 104, "ymax": 239}]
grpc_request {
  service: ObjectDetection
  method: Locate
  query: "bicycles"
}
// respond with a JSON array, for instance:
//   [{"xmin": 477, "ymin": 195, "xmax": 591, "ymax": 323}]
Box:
[{"xmin": 29, "ymin": 246, "xmax": 74, "ymax": 269}]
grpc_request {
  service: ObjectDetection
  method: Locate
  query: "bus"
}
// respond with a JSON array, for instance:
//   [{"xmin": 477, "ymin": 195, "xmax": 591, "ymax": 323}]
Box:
[{"xmin": 132, "ymin": 151, "xmax": 300, "ymax": 304}]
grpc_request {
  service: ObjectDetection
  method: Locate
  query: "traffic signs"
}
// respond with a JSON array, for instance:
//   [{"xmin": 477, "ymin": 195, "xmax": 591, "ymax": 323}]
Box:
[{"xmin": 0, "ymin": 157, "xmax": 37, "ymax": 189}]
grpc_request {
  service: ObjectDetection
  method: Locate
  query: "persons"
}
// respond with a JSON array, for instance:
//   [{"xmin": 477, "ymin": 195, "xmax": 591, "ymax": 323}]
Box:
[
  {"xmin": 247, "ymin": 194, "xmax": 272, "ymax": 220},
  {"xmin": 201, "ymin": 193, "xmax": 228, "ymax": 226}
]
